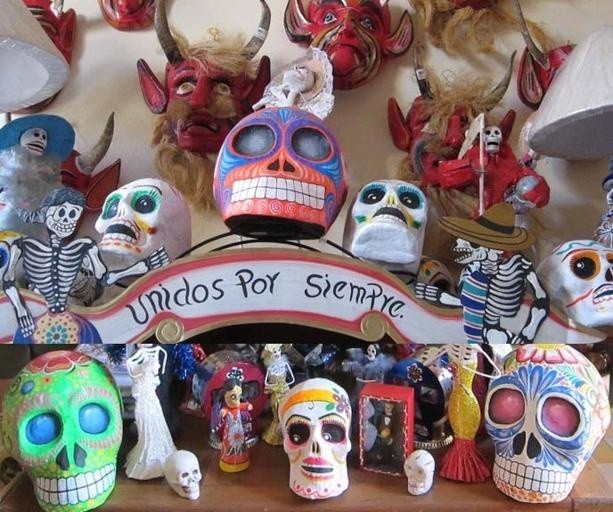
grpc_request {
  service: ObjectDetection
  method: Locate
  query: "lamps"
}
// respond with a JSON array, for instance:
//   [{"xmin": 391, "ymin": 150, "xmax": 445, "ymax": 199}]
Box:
[
  {"xmin": 0, "ymin": 0, "xmax": 72, "ymax": 113},
  {"xmin": 527, "ymin": 23, "xmax": 613, "ymax": 163}
]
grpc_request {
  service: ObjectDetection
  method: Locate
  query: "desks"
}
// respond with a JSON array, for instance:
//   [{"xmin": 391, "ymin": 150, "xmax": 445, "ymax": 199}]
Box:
[{"xmin": 1, "ymin": 413, "xmax": 612, "ymax": 512}]
